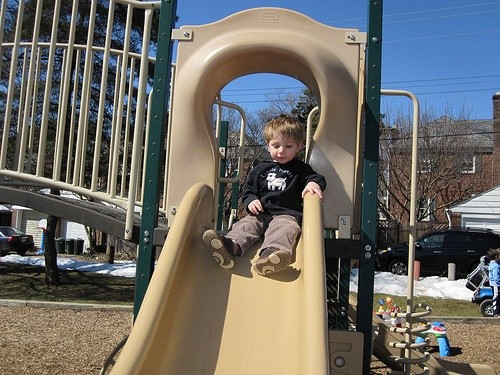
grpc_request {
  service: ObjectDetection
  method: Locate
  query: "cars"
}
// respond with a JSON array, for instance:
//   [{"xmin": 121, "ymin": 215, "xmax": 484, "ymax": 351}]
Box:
[{"xmin": 0, "ymin": 225, "xmax": 34, "ymax": 257}]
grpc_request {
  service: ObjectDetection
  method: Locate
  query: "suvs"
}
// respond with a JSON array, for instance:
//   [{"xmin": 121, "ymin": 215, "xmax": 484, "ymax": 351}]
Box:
[
  {"xmin": 375, "ymin": 227, "xmax": 499, "ymax": 277},
  {"xmin": 471, "ymin": 286, "xmax": 494, "ymax": 317}
]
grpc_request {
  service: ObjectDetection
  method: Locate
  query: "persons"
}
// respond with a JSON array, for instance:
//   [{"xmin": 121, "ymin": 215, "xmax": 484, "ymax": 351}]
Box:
[
  {"xmin": 202, "ymin": 115, "xmax": 326, "ymax": 276},
  {"xmin": 488, "ymin": 249, "xmax": 500, "ymax": 317}
]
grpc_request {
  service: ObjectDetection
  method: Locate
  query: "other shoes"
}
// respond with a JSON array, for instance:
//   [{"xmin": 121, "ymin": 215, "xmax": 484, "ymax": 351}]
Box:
[
  {"xmin": 253, "ymin": 247, "xmax": 291, "ymax": 276},
  {"xmin": 202, "ymin": 229, "xmax": 235, "ymax": 269}
]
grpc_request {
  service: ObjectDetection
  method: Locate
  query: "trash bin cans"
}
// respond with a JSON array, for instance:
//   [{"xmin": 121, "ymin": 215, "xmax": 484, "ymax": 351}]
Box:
[
  {"xmin": 73, "ymin": 238, "xmax": 84, "ymax": 255},
  {"xmin": 55, "ymin": 238, "xmax": 65, "ymax": 253},
  {"xmin": 65, "ymin": 238, "xmax": 74, "ymax": 253}
]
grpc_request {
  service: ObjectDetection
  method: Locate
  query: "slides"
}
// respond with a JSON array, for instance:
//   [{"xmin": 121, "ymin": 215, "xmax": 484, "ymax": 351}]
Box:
[
  {"xmin": 348, "ymin": 293, "xmax": 496, "ymax": 375},
  {"xmin": 102, "ymin": 182, "xmax": 332, "ymax": 375}
]
